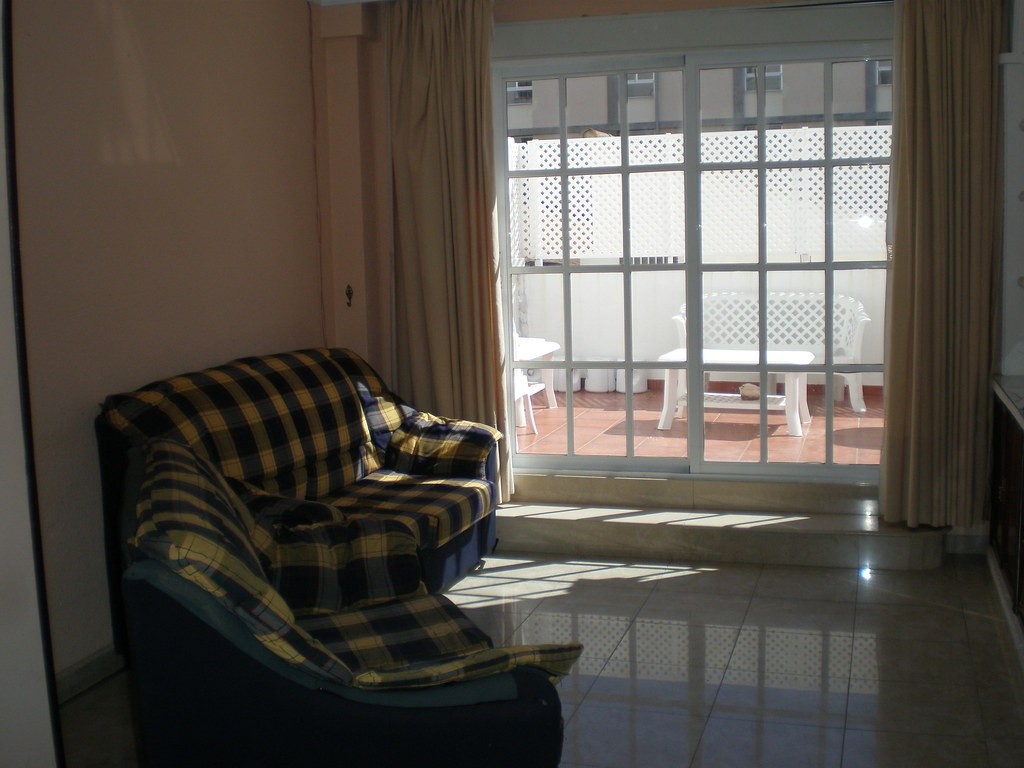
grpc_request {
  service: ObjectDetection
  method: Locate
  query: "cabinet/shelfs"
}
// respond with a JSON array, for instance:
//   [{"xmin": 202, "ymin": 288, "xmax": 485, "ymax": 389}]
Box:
[{"xmin": 990, "ymin": 374, "xmax": 1024, "ymax": 633}]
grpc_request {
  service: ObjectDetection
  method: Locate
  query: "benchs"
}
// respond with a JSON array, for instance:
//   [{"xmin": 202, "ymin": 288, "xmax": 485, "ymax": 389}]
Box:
[{"xmin": 672, "ymin": 290, "xmax": 872, "ymax": 413}]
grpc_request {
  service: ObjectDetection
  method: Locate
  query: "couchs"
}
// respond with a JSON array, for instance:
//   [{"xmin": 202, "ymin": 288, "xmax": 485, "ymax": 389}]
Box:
[
  {"xmin": 117, "ymin": 436, "xmax": 583, "ymax": 768},
  {"xmin": 94, "ymin": 348, "xmax": 506, "ymax": 662}
]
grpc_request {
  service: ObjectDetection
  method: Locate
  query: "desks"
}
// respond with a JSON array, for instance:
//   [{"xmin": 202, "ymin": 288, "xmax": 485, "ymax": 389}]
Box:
[
  {"xmin": 657, "ymin": 348, "xmax": 815, "ymax": 437},
  {"xmin": 516, "ymin": 337, "xmax": 562, "ymax": 428}
]
